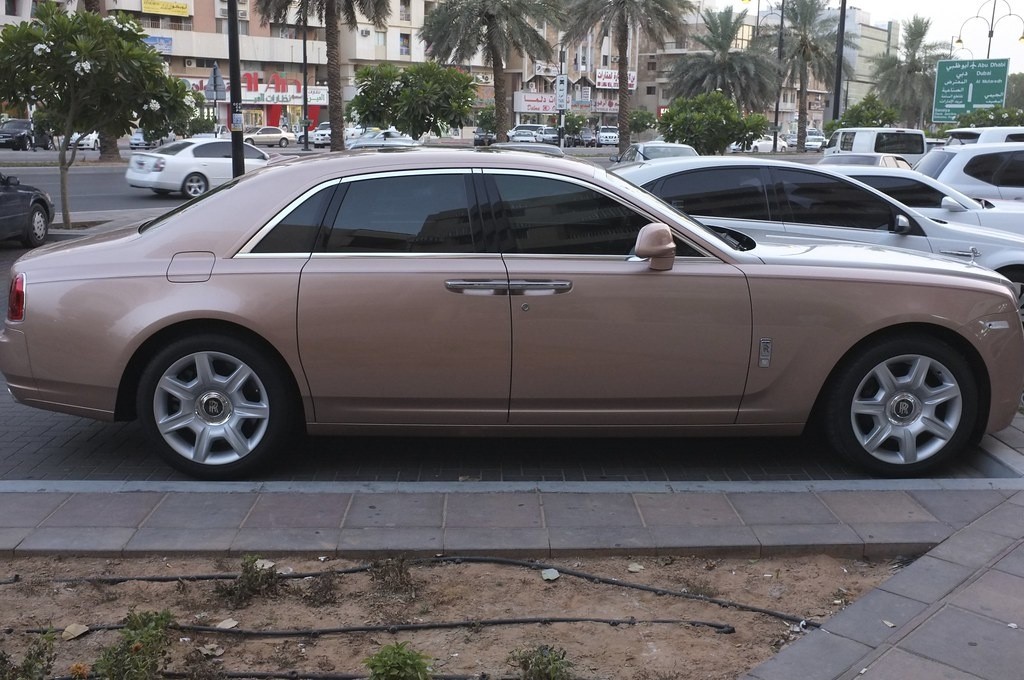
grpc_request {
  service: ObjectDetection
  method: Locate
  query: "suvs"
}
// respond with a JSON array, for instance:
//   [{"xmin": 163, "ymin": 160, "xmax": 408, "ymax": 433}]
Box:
[
  {"xmin": 534, "ymin": 125, "xmax": 619, "ymax": 148},
  {"xmin": 314, "ymin": 122, "xmax": 345, "ymax": 148}
]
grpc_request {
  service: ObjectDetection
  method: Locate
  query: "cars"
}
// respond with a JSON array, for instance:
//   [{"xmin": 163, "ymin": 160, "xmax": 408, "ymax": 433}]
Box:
[
  {"xmin": 125, "ymin": 139, "xmax": 270, "ymax": 200},
  {"xmin": 0, "ymin": 118, "xmax": 52, "ymax": 151},
  {"xmin": 129, "ymin": 126, "xmax": 175, "ymax": 150},
  {"xmin": 60, "ymin": 130, "xmax": 101, "ymax": 151},
  {"xmin": 653, "ymin": 109, "xmax": 828, "ymax": 154},
  {"xmin": 297, "ymin": 124, "xmax": 431, "ymax": 151},
  {"xmin": 510, "ymin": 130, "xmax": 537, "ymax": 142},
  {"xmin": 243, "ymin": 126, "xmax": 294, "ymax": 148},
  {"xmin": 0, "ymin": 170, "xmax": 56, "ymax": 249},
  {"xmin": 1, "ymin": 144, "xmax": 1024, "ymax": 480},
  {"xmin": 191, "ymin": 124, "xmax": 232, "ymax": 139},
  {"xmin": 472, "ymin": 128, "xmax": 497, "ymax": 146},
  {"xmin": 606, "ymin": 127, "xmax": 1024, "ymax": 329}
]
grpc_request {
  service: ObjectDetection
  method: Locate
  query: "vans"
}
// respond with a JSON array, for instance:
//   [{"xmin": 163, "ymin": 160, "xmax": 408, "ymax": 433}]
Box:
[{"xmin": 507, "ymin": 124, "xmax": 549, "ymax": 142}]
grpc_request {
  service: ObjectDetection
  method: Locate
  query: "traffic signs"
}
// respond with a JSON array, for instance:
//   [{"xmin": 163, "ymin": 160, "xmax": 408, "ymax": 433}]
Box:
[{"xmin": 932, "ymin": 59, "xmax": 1009, "ymax": 122}]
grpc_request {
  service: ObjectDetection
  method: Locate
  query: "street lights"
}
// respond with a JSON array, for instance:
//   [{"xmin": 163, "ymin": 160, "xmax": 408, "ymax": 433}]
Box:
[
  {"xmin": 955, "ymin": 0, "xmax": 1024, "ymax": 59},
  {"xmin": 543, "ymin": 42, "xmax": 578, "ymax": 147}
]
